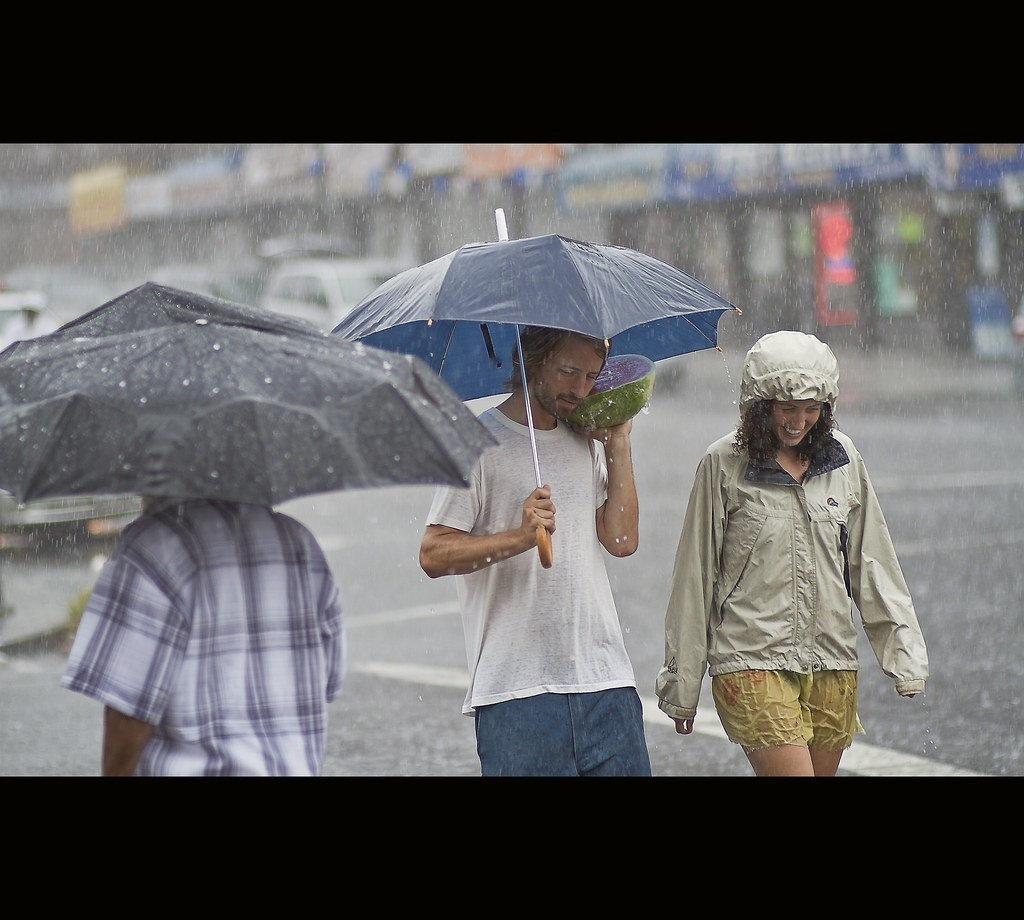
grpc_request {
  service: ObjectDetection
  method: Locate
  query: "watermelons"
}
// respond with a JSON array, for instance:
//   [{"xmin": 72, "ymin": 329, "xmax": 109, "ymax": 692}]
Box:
[{"xmin": 569, "ymin": 354, "xmax": 656, "ymax": 427}]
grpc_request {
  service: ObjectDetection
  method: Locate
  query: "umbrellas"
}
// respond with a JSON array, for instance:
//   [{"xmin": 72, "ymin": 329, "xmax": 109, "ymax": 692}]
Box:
[
  {"xmin": 326, "ymin": 207, "xmax": 744, "ymax": 570},
  {"xmin": 0, "ymin": 281, "xmax": 503, "ymax": 511}
]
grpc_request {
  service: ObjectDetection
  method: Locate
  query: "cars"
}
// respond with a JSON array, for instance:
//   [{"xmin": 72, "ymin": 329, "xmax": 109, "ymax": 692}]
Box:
[{"xmin": 1, "ymin": 249, "xmax": 418, "ymax": 535}]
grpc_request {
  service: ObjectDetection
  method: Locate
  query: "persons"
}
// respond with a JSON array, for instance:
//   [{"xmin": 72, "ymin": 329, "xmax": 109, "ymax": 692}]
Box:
[
  {"xmin": 418, "ymin": 324, "xmax": 654, "ymax": 777},
  {"xmin": 61, "ymin": 494, "xmax": 348, "ymax": 776},
  {"xmin": 654, "ymin": 327, "xmax": 930, "ymax": 776}
]
